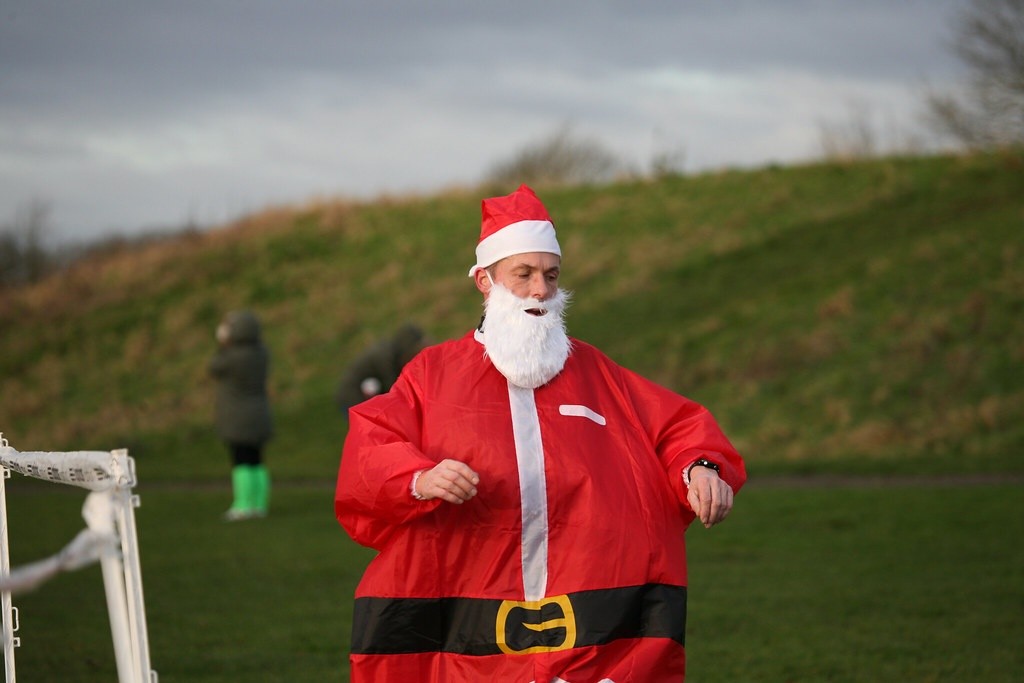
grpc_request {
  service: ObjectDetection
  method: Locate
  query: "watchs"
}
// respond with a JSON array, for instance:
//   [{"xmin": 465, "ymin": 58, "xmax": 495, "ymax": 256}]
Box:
[{"xmin": 688, "ymin": 459, "xmax": 720, "ymax": 483}]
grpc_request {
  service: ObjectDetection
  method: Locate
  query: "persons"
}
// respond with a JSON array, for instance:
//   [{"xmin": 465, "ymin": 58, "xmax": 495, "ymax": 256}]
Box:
[
  {"xmin": 210, "ymin": 310, "xmax": 271, "ymax": 521},
  {"xmin": 342, "ymin": 323, "xmax": 425, "ymax": 425},
  {"xmin": 333, "ymin": 185, "xmax": 748, "ymax": 683}
]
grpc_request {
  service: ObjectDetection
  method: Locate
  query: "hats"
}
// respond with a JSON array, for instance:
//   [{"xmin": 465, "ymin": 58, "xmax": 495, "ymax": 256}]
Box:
[{"xmin": 471, "ymin": 187, "xmax": 561, "ymax": 273}]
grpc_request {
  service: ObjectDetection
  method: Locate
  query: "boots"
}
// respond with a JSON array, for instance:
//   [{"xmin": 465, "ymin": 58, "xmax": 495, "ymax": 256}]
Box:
[{"xmin": 224, "ymin": 464, "xmax": 268, "ymax": 520}]
